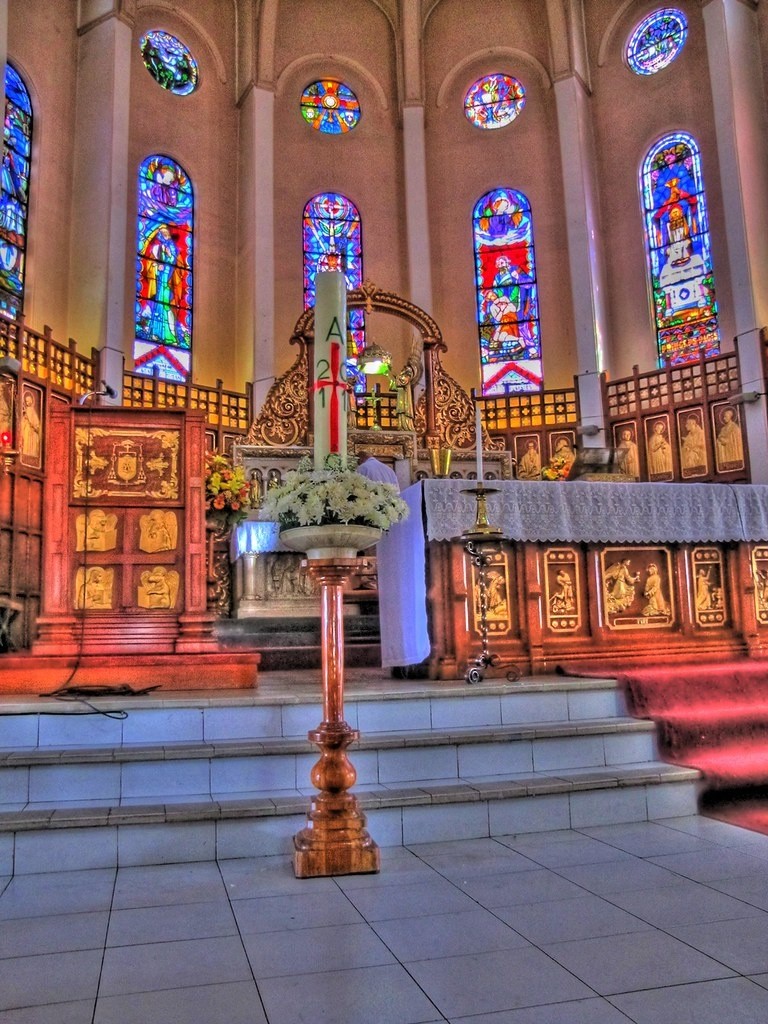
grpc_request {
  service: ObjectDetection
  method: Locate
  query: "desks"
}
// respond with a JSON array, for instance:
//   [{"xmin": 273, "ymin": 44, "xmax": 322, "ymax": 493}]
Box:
[{"xmin": 376, "ymin": 479, "xmax": 768, "ymax": 682}]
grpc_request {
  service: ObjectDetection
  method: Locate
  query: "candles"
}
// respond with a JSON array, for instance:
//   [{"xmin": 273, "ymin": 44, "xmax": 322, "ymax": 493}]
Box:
[
  {"xmin": 312, "ymin": 270, "xmax": 349, "ymax": 472},
  {"xmin": 475, "ymin": 409, "xmax": 483, "ymax": 482}
]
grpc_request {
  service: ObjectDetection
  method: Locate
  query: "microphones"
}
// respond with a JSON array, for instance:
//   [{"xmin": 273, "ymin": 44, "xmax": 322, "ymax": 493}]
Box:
[{"xmin": 100, "ymin": 380, "xmax": 117, "ymax": 399}]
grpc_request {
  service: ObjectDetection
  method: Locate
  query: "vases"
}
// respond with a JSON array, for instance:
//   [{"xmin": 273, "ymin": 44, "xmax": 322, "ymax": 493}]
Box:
[{"xmin": 281, "ymin": 523, "xmax": 383, "ymax": 560}]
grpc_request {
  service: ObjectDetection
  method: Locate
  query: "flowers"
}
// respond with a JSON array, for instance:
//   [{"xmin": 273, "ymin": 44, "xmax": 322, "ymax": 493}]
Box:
[
  {"xmin": 204, "ymin": 453, "xmax": 261, "ymax": 527},
  {"xmin": 259, "ymin": 454, "xmax": 408, "ymax": 532}
]
